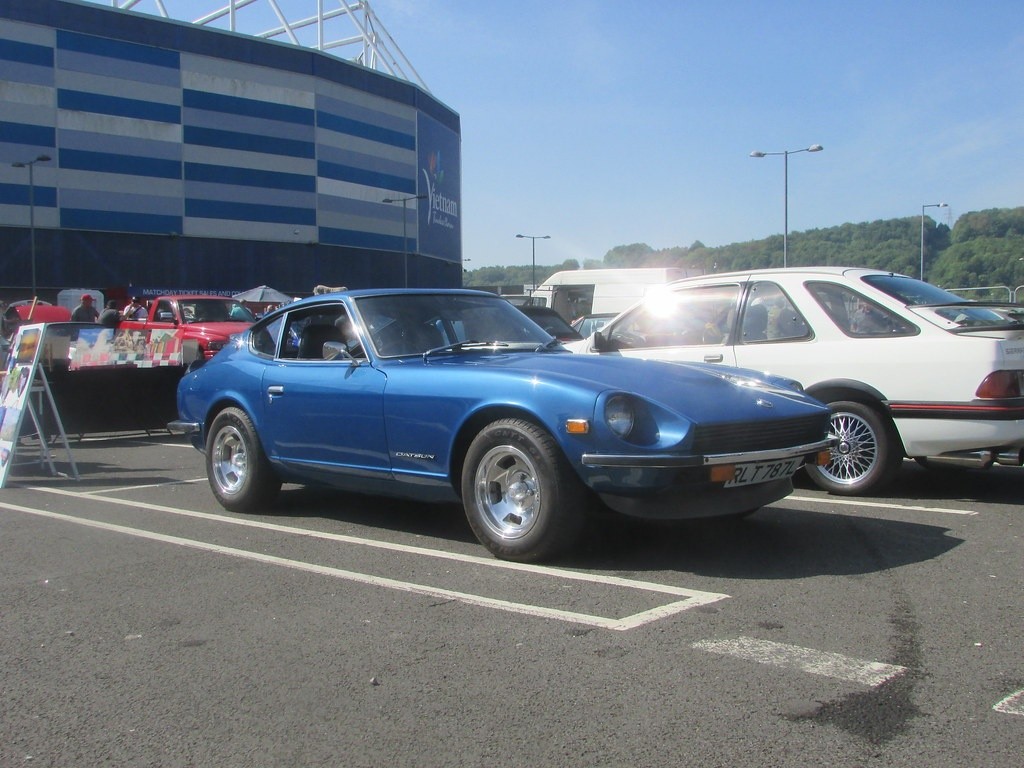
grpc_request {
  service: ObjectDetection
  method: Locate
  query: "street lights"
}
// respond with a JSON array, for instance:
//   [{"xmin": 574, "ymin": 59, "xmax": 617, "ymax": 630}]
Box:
[
  {"xmin": 12, "ymin": 154, "xmax": 53, "ymax": 298},
  {"xmin": 749, "ymin": 145, "xmax": 825, "ymax": 268},
  {"xmin": 515, "ymin": 233, "xmax": 551, "ymax": 292},
  {"xmin": 381, "ymin": 194, "xmax": 428, "ymax": 288},
  {"xmin": 920, "ymin": 203, "xmax": 949, "ymax": 281}
]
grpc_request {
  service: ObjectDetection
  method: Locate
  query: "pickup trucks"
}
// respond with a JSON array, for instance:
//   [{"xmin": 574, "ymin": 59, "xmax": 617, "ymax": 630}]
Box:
[{"xmin": 117, "ymin": 292, "xmax": 258, "ymax": 361}]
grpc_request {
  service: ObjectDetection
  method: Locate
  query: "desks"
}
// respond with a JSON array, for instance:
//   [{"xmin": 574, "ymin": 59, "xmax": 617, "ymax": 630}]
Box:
[{"xmin": 18, "ymin": 364, "xmax": 188, "ymax": 445}]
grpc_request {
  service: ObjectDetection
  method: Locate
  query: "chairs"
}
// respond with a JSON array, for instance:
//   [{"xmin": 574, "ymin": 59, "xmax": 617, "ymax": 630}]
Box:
[
  {"xmin": 296, "ymin": 322, "xmax": 447, "ymax": 360},
  {"xmin": 194, "ymin": 301, "xmax": 231, "ymax": 322},
  {"xmin": 720, "ymin": 305, "xmax": 810, "ymax": 344}
]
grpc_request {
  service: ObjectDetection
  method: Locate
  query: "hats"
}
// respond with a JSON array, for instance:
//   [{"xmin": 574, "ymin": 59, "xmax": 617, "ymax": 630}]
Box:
[
  {"xmin": 131, "ymin": 297, "xmax": 139, "ymax": 303},
  {"xmin": 107, "ymin": 300, "xmax": 118, "ymax": 306},
  {"xmin": 81, "ymin": 294, "xmax": 96, "ymax": 301}
]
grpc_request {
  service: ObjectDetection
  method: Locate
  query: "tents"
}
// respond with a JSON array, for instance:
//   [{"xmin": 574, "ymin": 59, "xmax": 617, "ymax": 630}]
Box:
[{"xmin": 230, "ymin": 284, "xmax": 294, "ymax": 303}]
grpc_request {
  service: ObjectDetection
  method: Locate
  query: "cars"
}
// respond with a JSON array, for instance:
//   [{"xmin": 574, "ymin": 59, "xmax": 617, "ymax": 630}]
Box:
[
  {"xmin": 0, "ymin": 300, "xmax": 72, "ymax": 371},
  {"xmin": 441, "ymin": 305, "xmax": 583, "ymax": 347},
  {"xmin": 561, "ymin": 266, "xmax": 1023, "ymax": 495},
  {"xmin": 570, "ymin": 312, "xmax": 622, "ymax": 338},
  {"xmin": 166, "ymin": 289, "xmax": 842, "ymax": 565}
]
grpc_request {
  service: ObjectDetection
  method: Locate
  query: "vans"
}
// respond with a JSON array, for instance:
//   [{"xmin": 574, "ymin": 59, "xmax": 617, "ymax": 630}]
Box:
[{"xmin": 523, "ymin": 266, "xmax": 688, "ymax": 331}]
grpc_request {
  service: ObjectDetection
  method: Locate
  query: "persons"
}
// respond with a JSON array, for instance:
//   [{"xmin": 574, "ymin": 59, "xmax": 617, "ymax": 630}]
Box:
[
  {"xmin": 98, "ymin": 300, "xmax": 123, "ymax": 321},
  {"xmin": 0, "ymin": 300, "xmax": 19, "ymax": 333},
  {"xmin": 850, "ymin": 301, "xmax": 883, "ymax": 332},
  {"xmin": 262, "ymin": 305, "xmax": 277, "ymax": 317},
  {"xmin": 231, "ymin": 299, "xmax": 252, "ymax": 321},
  {"xmin": 570, "ymin": 297, "xmax": 579, "ymax": 320},
  {"xmin": 123, "ymin": 297, "xmax": 147, "ymax": 321},
  {"xmin": 72, "ymin": 294, "xmax": 99, "ymax": 321}
]
